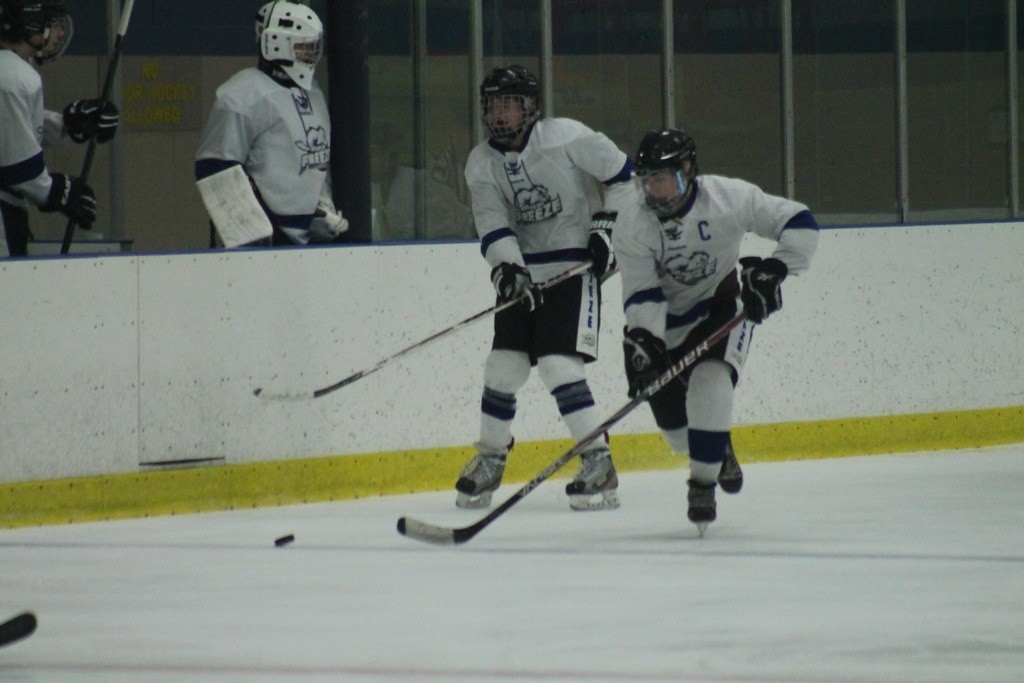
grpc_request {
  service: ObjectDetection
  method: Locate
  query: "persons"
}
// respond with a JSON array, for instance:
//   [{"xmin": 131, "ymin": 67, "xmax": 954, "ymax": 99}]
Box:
[
  {"xmin": 455, "ymin": 65, "xmax": 636, "ymax": 511},
  {"xmin": 0, "ymin": 0, "xmax": 120, "ymax": 259},
  {"xmin": 195, "ymin": 0, "xmax": 349, "ymax": 246},
  {"xmin": 612, "ymin": 130, "xmax": 822, "ymax": 537}
]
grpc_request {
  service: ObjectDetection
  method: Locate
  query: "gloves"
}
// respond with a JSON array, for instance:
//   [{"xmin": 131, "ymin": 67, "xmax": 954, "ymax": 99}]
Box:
[
  {"xmin": 37, "ymin": 172, "xmax": 96, "ymax": 230},
  {"xmin": 585, "ymin": 210, "xmax": 618, "ymax": 278},
  {"xmin": 491, "ymin": 261, "xmax": 545, "ymax": 314},
  {"xmin": 63, "ymin": 98, "xmax": 119, "ymax": 144},
  {"xmin": 738, "ymin": 255, "xmax": 789, "ymax": 324},
  {"xmin": 622, "ymin": 324, "xmax": 676, "ymax": 399}
]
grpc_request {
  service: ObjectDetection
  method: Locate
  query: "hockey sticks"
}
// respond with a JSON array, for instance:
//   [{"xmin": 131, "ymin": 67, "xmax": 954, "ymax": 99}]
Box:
[
  {"xmin": 254, "ymin": 260, "xmax": 593, "ymax": 402},
  {"xmin": 0, "ymin": 614, "xmax": 36, "ymax": 646},
  {"xmin": 60, "ymin": 0, "xmax": 135, "ymax": 255},
  {"xmin": 397, "ymin": 311, "xmax": 747, "ymax": 545}
]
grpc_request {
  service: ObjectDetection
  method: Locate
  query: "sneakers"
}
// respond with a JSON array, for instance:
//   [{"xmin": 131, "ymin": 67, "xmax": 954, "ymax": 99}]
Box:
[
  {"xmin": 718, "ymin": 436, "xmax": 743, "ymax": 493},
  {"xmin": 686, "ymin": 478, "xmax": 718, "ymax": 537},
  {"xmin": 564, "ymin": 430, "xmax": 619, "ymax": 510},
  {"xmin": 454, "ymin": 435, "xmax": 515, "ymax": 510}
]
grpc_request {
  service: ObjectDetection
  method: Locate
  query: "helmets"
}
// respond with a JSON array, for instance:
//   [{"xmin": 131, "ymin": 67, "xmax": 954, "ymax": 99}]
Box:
[
  {"xmin": 0, "ymin": 0, "xmax": 75, "ymax": 68},
  {"xmin": 481, "ymin": 64, "xmax": 540, "ymax": 145},
  {"xmin": 635, "ymin": 129, "xmax": 697, "ymax": 217},
  {"xmin": 255, "ymin": 1, "xmax": 326, "ymax": 91}
]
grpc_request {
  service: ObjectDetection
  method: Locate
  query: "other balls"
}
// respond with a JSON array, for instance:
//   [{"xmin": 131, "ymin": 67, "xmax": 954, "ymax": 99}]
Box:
[{"xmin": 275, "ymin": 536, "xmax": 294, "ymax": 545}]
